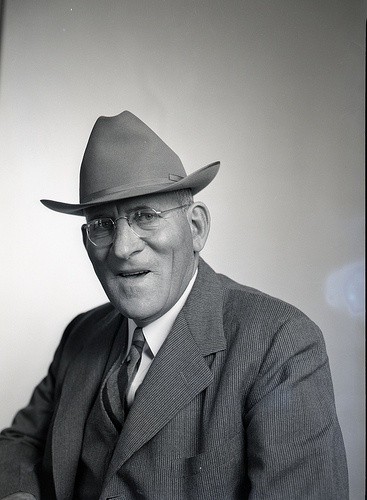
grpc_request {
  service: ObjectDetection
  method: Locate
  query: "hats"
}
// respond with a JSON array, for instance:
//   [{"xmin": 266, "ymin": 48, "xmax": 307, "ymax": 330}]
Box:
[{"xmin": 40, "ymin": 111, "xmax": 221, "ymax": 216}]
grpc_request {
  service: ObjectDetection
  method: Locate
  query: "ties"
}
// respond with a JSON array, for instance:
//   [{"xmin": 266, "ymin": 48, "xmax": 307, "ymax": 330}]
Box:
[{"xmin": 105, "ymin": 327, "xmax": 146, "ymax": 435}]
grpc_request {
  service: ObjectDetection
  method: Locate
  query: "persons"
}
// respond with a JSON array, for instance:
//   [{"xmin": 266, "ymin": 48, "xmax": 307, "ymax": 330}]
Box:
[{"xmin": 0, "ymin": 111, "xmax": 350, "ymax": 499}]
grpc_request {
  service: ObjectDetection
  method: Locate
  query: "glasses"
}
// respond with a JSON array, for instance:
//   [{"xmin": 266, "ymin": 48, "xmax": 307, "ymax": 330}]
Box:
[{"xmin": 84, "ymin": 204, "xmax": 189, "ymax": 247}]
